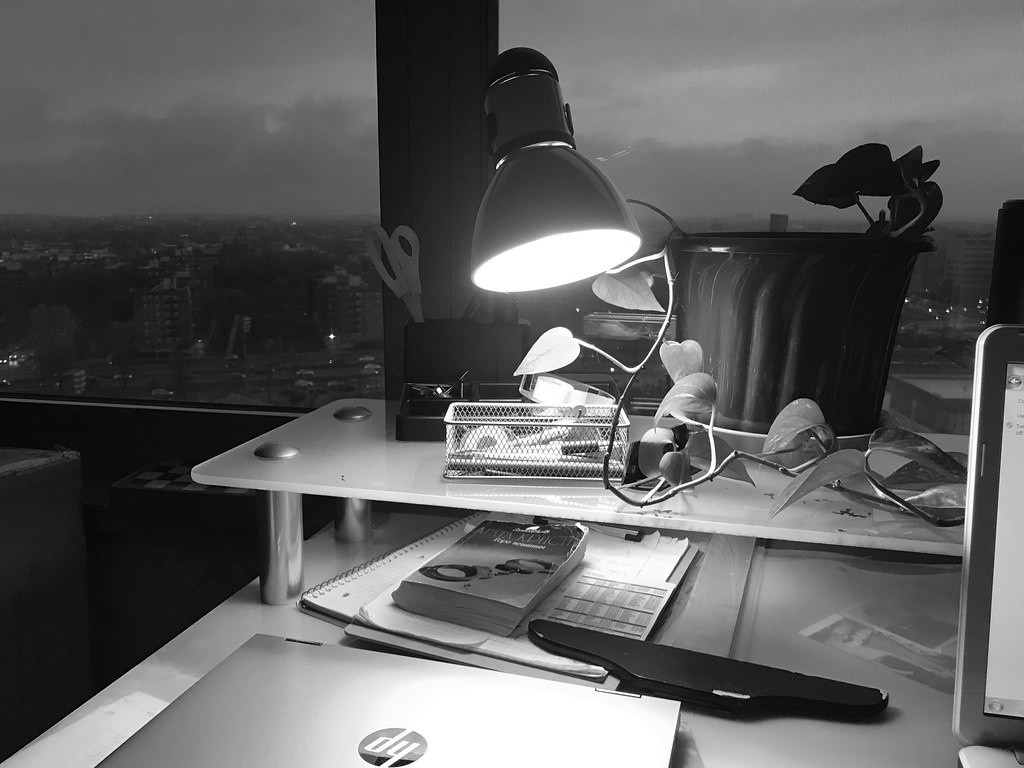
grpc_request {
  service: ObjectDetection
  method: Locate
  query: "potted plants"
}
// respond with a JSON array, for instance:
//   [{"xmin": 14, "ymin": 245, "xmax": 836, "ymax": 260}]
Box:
[{"xmin": 502, "ymin": 142, "xmax": 967, "ymax": 519}]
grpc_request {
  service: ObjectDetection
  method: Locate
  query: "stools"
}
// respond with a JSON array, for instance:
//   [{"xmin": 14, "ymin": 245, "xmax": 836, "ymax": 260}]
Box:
[{"xmin": 93, "ymin": 458, "xmax": 263, "ymax": 658}]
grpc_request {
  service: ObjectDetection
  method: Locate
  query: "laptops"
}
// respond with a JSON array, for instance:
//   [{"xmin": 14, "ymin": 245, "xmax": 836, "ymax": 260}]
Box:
[
  {"xmin": 90, "ymin": 634, "xmax": 683, "ymax": 768},
  {"xmin": 953, "ymin": 324, "xmax": 1024, "ymax": 768}
]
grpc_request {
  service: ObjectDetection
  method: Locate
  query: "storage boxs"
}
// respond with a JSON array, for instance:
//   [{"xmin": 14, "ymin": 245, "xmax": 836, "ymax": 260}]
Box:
[{"xmin": 1, "ymin": 446, "xmax": 91, "ymax": 762}]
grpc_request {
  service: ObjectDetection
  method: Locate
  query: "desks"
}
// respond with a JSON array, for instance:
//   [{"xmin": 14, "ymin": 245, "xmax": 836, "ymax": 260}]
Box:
[{"xmin": 2, "ymin": 386, "xmax": 1024, "ymax": 768}]
